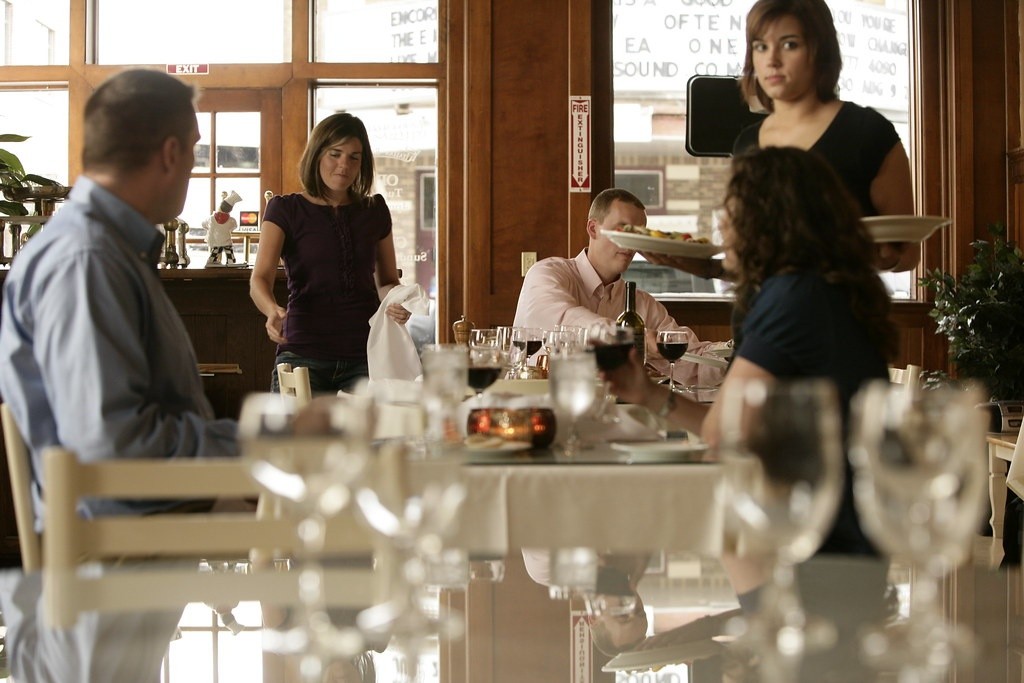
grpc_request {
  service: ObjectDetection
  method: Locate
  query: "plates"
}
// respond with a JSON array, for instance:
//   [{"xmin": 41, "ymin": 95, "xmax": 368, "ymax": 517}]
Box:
[
  {"xmin": 858, "ymin": 215, "xmax": 950, "ymax": 243},
  {"xmin": 610, "ymin": 442, "xmax": 709, "ymax": 463},
  {"xmin": 680, "ymin": 348, "xmax": 733, "ymax": 369},
  {"xmin": 600, "ymin": 229, "xmax": 725, "ymax": 260}
]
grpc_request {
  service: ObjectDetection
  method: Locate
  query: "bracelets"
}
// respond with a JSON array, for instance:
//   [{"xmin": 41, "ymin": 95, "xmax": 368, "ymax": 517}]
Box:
[{"xmin": 876, "ymin": 243, "xmax": 903, "ymax": 273}]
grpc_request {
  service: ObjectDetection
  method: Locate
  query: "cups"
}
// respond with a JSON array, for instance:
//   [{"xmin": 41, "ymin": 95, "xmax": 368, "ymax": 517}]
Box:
[
  {"xmin": 468, "ymin": 560, "xmax": 505, "ymax": 583},
  {"xmin": 547, "ymin": 325, "xmax": 588, "ymax": 352},
  {"xmin": 568, "ymin": 595, "xmax": 637, "ymax": 617}
]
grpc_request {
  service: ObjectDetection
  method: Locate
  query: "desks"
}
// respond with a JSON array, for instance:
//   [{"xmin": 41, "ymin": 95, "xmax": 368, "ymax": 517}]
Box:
[
  {"xmin": 439, "ymin": 466, "xmax": 719, "ymax": 555},
  {"xmin": 984, "ymin": 433, "xmax": 1017, "ymax": 569},
  {"xmin": 0, "ymin": 557, "xmax": 1024, "ymax": 682}
]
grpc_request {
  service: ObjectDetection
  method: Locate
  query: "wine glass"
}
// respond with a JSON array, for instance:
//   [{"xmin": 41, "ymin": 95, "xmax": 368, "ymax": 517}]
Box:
[
  {"xmin": 548, "ymin": 350, "xmax": 597, "ymax": 458},
  {"xmin": 588, "ymin": 326, "xmax": 634, "ymax": 403},
  {"xmin": 419, "ymin": 327, "xmax": 503, "ymax": 442},
  {"xmin": 716, "ymin": 377, "xmax": 991, "ymax": 683},
  {"xmin": 656, "ymin": 331, "xmax": 688, "ymax": 393},
  {"xmin": 497, "ymin": 325, "xmax": 544, "ymax": 380},
  {"xmin": 237, "ymin": 389, "xmax": 469, "ymax": 653}
]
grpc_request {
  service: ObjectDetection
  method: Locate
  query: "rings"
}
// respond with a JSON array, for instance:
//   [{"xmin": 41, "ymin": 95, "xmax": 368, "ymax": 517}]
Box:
[{"xmin": 406, "ymin": 314, "xmax": 410, "ymax": 320}]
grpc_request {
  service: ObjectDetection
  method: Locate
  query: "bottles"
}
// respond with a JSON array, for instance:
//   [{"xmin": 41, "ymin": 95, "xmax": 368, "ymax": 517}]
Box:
[{"xmin": 615, "ymin": 281, "xmax": 644, "ymax": 367}]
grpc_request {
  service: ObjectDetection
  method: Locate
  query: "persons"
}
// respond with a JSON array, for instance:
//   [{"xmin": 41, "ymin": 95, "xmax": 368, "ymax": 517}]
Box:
[
  {"xmin": 636, "ymin": 0, "xmax": 922, "ymax": 285},
  {"xmin": 256, "ymin": 593, "xmax": 400, "ymax": 683},
  {"xmin": 0, "ymin": 68, "xmax": 359, "ymax": 563},
  {"xmin": 507, "ymin": 185, "xmax": 734, "ymax": 387},
  {"xmin": 520, "ymin": 543, "xmax": 657, "ymax": 658},
  {"xmin": 249, "ymin": 112, "xmax": 413, "ymax": 507},
  {"xmin": 697, "ymin": 143, "xmax": 899, "ymax": 556}
]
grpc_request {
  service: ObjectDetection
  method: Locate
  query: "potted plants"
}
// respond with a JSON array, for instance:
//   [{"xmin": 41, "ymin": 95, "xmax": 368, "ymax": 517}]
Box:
[{"xmin": 920, "ymin": 237, "xmax": 1024, "ymax": 432}]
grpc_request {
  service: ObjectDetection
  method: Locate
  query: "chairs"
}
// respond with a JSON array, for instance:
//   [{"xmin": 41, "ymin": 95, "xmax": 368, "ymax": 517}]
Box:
[{"xmin": 0, "ymin": 361, "xmax": 421, "ymax": 568}]
